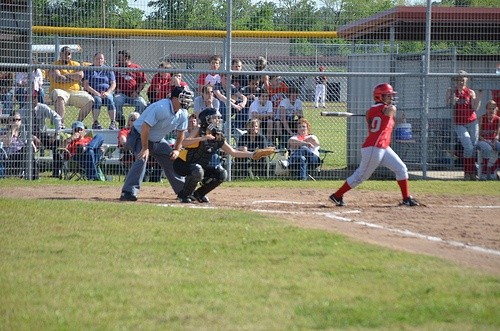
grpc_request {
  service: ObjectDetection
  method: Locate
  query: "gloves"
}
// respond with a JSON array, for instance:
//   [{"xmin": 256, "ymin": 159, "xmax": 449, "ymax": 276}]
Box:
[{"xmin": 252, "ymin": 147, "xmax": 276, "ymax": 160}]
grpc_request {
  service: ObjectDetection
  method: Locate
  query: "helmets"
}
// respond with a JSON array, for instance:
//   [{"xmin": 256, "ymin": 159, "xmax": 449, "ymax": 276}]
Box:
[
  {"xmin": 198, "ymin": 108, "xmax": 223, "ymax": 134},
  {"xmin": 373, "ymin": 83, "xmax": 398, "ymax": 105}
]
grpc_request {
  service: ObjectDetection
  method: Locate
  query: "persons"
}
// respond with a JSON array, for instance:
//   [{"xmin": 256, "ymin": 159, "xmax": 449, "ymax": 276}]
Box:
[
  {"xmin": 0, "ymin": 46, "xmax": 172, "ymax": 183},
  {"xmin": 476, "ymin": 100, "xmax": 500, "ymax": 181},
  {"xmin": 328, "ymin": 83, "xmax": 421, "ymax": 206},
  {"xmin": 447, "ymin": 70, "xmax": 481, "ymax": 181},
  {"xmin": 120, "ymin": 86, "xmax": 198, "ymax": 203},
  {"xmin": 173, "ymin": 108, "xmax": 276, "ymax": 203},
  {"xmin": 158, "ymin": 54, "xmax": 327, "ymax": 181}
]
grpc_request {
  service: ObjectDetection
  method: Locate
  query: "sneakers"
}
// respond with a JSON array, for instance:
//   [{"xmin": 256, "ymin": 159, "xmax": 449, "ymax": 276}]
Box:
[
  {"xmin": 480, "ymin": 173, "xmax": 487, "ymax": 180},
  {"xmin": 402, "ymin": 195, "xmax": 420, "ymax": 207},
  {"xmin": 329, "ymin": 193, "xmax": 348, "ymax": 206},
  {"xmin": 92, "ymin": 122, "xmax": 103, "ymax": 129},
  {"xmin": 487, "ymin": 174, "xmax": 496, "ymax": 179},
  {"xmin": 464, "ymin": 174, "xmax": 471, "ymax": 181},
  {"xmin": 471, "ymin": 175, "xmax": 480, "ymax": 180},
  {"xmin": 109, "ymin": 121, "xmax": 117, "ymax": 130}
]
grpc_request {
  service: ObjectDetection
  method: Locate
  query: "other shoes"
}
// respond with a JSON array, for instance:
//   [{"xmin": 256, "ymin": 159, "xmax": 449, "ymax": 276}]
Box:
[
  {"xmin": 277, "ymin": 160, "xmax": 288, "ymax": 170},
  {"xmin": 76, "ymin": 144, "xmax": 84, "ymax": 156},
  {"xmin": 189, "ymin": 195, "xmax": 196, "ymax": 201},
  {"xmin": 61, "ymin": 123, "xmax": 66, "ymax": 129},
  {"xmin": 118, "ymin": 120, "xmax": 125, "ymax": 129},
  {"xmin": 120, "ymin": 190, "xmax": 137, "ymax": 201},
  {"xmin": 193, "ymin": 191, "xmax": 210, "ymax": 203},
  {"xmin": 176, "ymin": 195, "xmax": 191, "ymax": 203}
]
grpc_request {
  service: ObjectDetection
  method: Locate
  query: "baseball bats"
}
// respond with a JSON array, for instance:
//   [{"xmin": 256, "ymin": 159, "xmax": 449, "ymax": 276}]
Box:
[{"xmin": 321, "ymin": 111, "xmax": 366, "ymax": 117}]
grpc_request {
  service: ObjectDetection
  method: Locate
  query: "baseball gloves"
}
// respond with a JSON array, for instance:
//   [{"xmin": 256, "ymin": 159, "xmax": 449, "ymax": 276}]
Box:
[{"xmin": 252, "ymin": 148, "xmax": 275, "ymax": 161}]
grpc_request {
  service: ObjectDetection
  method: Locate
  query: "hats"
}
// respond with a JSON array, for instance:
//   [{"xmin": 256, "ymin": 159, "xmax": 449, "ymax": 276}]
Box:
[
  {"xmin": 72, "ymin": 121, "xmax": 84, "ymax": 129},
  {"xmin": 25, "ymin": 90, "xmax": 38, "ymax": 96},
  {"xmin": 188, "ymin": 114, "xmax": 198, "ymax": 119}
]
